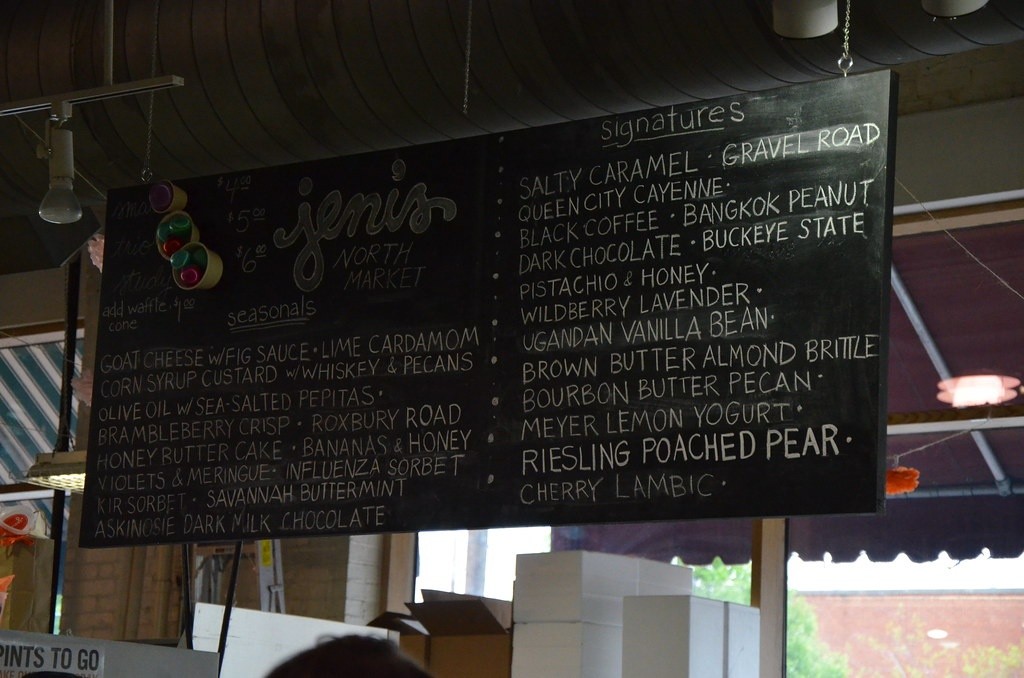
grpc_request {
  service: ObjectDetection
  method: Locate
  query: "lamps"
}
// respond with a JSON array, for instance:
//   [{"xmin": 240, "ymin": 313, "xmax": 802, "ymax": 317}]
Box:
[
  {"xmin": 15, "ymin": 450, "xmax": 88, "ymax": 496},
  {"xmin": 917, "ymin": 0, "xmax": 990, "ymax": 20},
  {"xmin": 771, "ymin": 0, "xmax": 842, "ymax": 43},
  {"xmin": 34, "ymin": 101, "xmax": 84, "ymax": 223}
]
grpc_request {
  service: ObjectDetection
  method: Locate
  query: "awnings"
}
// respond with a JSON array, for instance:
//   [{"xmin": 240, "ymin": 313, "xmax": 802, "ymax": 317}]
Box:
[
  {"xmin": 0, "ymin": 337, "xmax": 96, "ymax": 538},
  {"xmin": 554, "ymin": 220, "xmax": 1023, "ymax": 563}
]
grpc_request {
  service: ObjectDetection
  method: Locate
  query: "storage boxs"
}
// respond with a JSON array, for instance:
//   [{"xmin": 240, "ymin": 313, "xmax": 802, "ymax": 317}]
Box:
[
  {"xmin": 368, "ymin": 588, "xmax": 512, "ymax": 678},
  {"xmin": 511, "ymin": 549, "xmax": 761, "ymax": 678}
]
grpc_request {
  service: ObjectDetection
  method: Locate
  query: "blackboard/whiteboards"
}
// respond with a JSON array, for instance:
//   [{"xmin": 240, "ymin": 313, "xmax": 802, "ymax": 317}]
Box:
[{"xmin": 74, "ymin": 65, "xmax": 900, "ymax": 550}]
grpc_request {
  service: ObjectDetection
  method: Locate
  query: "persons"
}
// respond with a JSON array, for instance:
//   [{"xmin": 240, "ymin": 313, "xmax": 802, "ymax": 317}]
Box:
[{"xmin": 265, "ymin": 634, "xmax": 431, "ymax": 678}]
